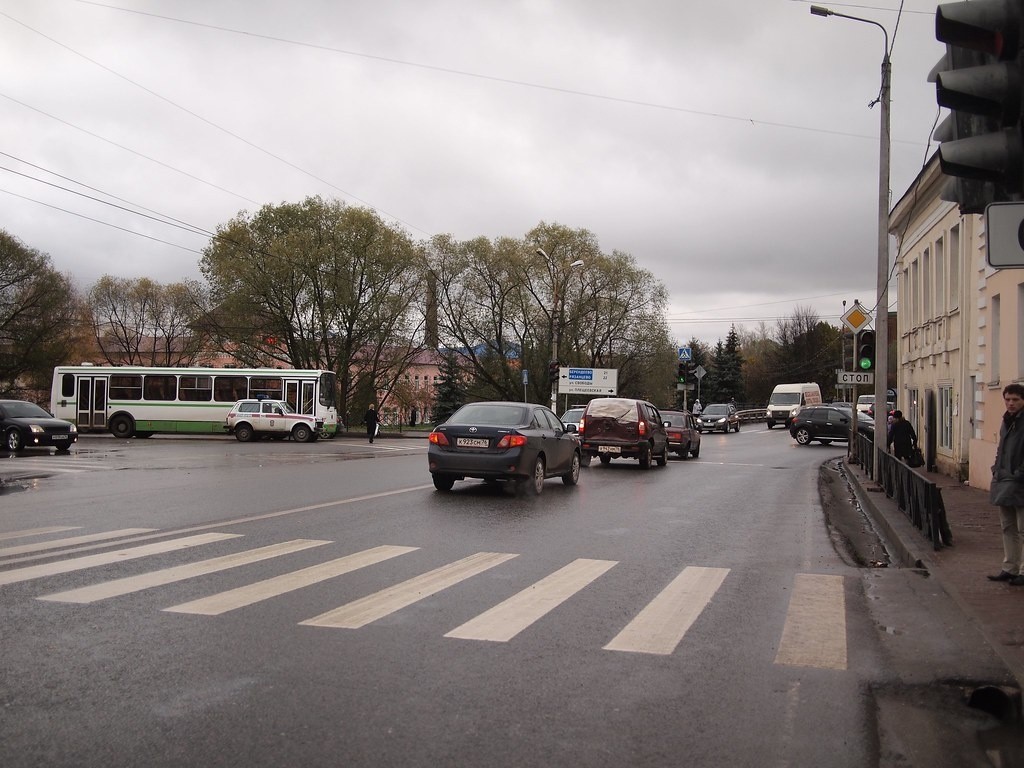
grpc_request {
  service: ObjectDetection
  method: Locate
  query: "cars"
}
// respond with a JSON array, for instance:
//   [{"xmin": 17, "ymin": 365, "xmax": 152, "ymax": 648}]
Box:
[
  {"xmin": 559, "ymin": 404, "xmax": 585, "ymax": 438},
  {"xmin": 657, "ymin": 408, "xmax": 702, "ymax": 461},
  {"xmin": 0, "ymin": 399, "xmax": 79, "ymax": 452},
  {"xmin": 827, "ymin": 392, "xmax": 896, "ymax": 424},
  {"xmin": 426, "ymin": 400, "xmax": 582, "ymax": 497}
]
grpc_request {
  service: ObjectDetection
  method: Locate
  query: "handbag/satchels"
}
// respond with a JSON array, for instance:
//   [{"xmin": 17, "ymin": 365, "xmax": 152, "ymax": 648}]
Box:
[{"xmin": 910, "ymin": 449, "xmax": 925, "ymax": 468}]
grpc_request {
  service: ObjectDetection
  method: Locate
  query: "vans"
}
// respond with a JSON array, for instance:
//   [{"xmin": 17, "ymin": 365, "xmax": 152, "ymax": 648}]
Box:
[{"xmin": 765, "ymin": 382, "xmax": 823, "ymax": 430}]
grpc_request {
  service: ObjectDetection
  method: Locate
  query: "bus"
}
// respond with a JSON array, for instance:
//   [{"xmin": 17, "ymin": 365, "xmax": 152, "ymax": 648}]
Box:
[{"xmin": 50, "ymin": 361, "xmax": 338, "ymax": 440}]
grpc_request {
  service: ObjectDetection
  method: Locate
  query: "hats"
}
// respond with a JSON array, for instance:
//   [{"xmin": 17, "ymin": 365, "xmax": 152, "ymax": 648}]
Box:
[{"xmin": 893, "ymin": 411, "xmax": 902, "ymax": 418}]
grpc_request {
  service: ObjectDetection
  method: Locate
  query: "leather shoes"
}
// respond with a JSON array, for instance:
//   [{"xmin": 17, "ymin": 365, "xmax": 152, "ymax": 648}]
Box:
[
  {"xmin": 986, "ymin": 570, "xmax": 1017, "ymax": 581},
  {"xmin": 1009, "ymin": 575, "xmax": 1024, "ymax": 586}
]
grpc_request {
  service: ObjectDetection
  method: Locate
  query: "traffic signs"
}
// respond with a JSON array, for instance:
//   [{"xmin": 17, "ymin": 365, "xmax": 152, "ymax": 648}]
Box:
[{"xmin": 557, "ymin": 366, "xmax": 617, "ymax": 396}]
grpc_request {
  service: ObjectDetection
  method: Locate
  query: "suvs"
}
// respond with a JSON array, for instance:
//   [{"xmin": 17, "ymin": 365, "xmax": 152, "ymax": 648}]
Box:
[
  {"xmin": 696, "ymin": 402, "xmax": 741, "ymax": 433},
  {"xmin": 789, "ymin": 403, "xmax": 875, "ymax": 446},
  {"xmin": 222, "ymin": 393, "xmax": 323, "ymax": 442},
  {"xmin": 577, "ymin": 397, "xmax": 672, "ymax": 470}
]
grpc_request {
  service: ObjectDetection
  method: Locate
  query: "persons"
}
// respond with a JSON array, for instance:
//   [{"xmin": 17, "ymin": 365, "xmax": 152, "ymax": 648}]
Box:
[
  {"xmin": 364, "ymin": 404, "xmax": 380, "ymax": 443},
  {"xmin": 731, "ymin": 398, "xmax": 736, "ymax": 409},
  {"xmin": 410, "ymin": 406, "xmax": 416, "ymax": 426},
  {"xmin": 987, "ymin": 385, "xmax": 1024, "ymax": 586},
  {"xmin": 692, "ymin": 399, "xmax": 702, "ymax": 414},
  {"xmin": 887, "ymin": 410, "xmax": 917, "ymax": 462}
]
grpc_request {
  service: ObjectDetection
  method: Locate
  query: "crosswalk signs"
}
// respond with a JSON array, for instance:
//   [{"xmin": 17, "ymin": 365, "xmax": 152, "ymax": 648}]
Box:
[
  {"xmin": 522, "ymin": 369, "xmax": 528, "ymax": 385},
  {"xmin": 678, "ymin": 348, "xmax": 692, "ymax": 361}
]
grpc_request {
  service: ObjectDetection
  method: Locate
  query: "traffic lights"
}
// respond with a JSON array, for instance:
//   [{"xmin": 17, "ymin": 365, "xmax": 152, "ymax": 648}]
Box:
[
  {"xmin": 926, "ymin": 42, "xmax": 1013, "ymax": 215},
  {"xmin": 934, "ymin": 0, "xmax": 1024, "ymax": 195},
  {"xmin": 857, "ymin": 329, "xmax": 876, "ymax": 372},
  {"xmin": 686, "ymin": 362, "xmax": 698, "ymax": 384},
  {"xmin": 843, "ymin": 332, "xmax": 857, "ymax": 373},
  {"xmin": 678, "ymin": 362, "xmax": 687, "ymax": 383},
  {"xmin": 549, "ymin": 361, "xmax": 560, "ymax": 383}
]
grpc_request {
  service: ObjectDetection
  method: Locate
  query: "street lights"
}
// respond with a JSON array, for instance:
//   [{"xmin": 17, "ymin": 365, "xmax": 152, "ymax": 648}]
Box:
[
  {"xmin": 809, "ymin": 4, "xmax": 892, "ymax": 485},
  {"xmin": 535, "ymin": 247, "xmax": 586, "ymax": 416}
]
